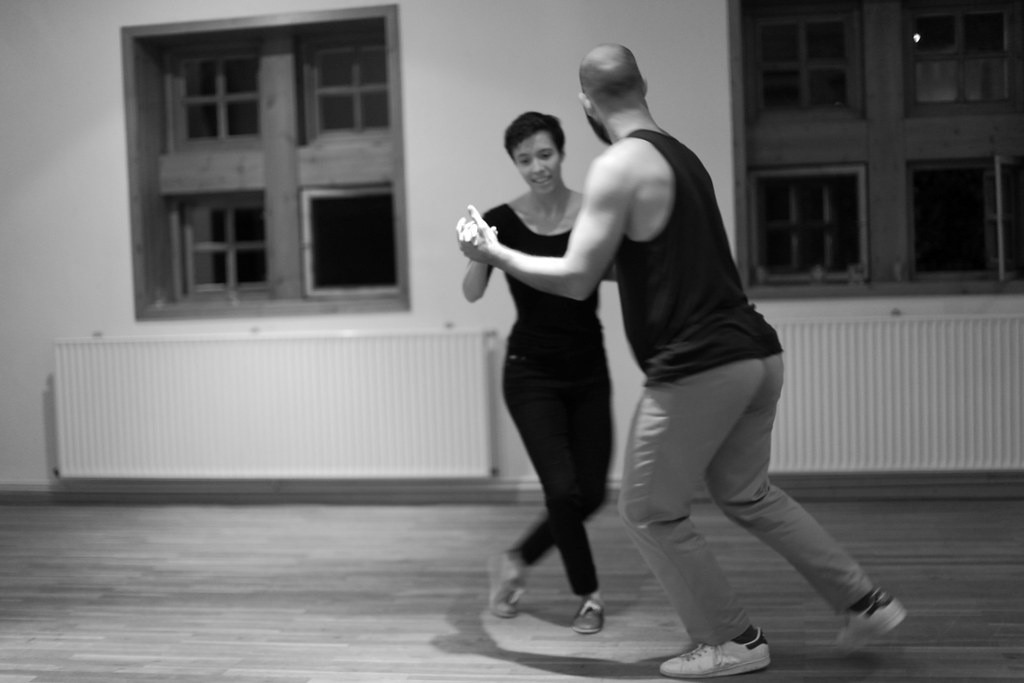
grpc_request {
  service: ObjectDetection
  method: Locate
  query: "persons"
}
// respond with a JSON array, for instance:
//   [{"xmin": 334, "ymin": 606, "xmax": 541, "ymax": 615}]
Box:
[
  {"xmin": 455, "ymin": 43, "xmax": 907, "ymax": 680},
  {"xmin": 460, "ymin": 111, "xmax": 614, "ymax": 635}
]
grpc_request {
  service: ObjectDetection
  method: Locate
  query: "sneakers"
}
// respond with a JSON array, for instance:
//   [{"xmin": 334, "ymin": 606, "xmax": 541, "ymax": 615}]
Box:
[
  {"xmin": 487, "ymin": 551, "xmax": 530, "ymax": 619},
  {"xmin": 660, "ymin": 627, "xmax": 770, "ymax": 678},
  {"xmin": 573, "ymin": 597, "xmax": 604, "ymax": 634},
  {"xmin": 838, "ymin": 587, "xmax": 908, "ymax": 657}
]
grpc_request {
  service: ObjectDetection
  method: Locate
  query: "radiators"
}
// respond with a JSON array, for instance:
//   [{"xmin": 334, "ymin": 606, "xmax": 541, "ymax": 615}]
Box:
[
  {"xmin": 57, "ymin": 328, "xmax": 501, "ymax": 478},
  {"xmin": 763, "ymin": 313, "xmax": 1024, "ymax": 474}
]
